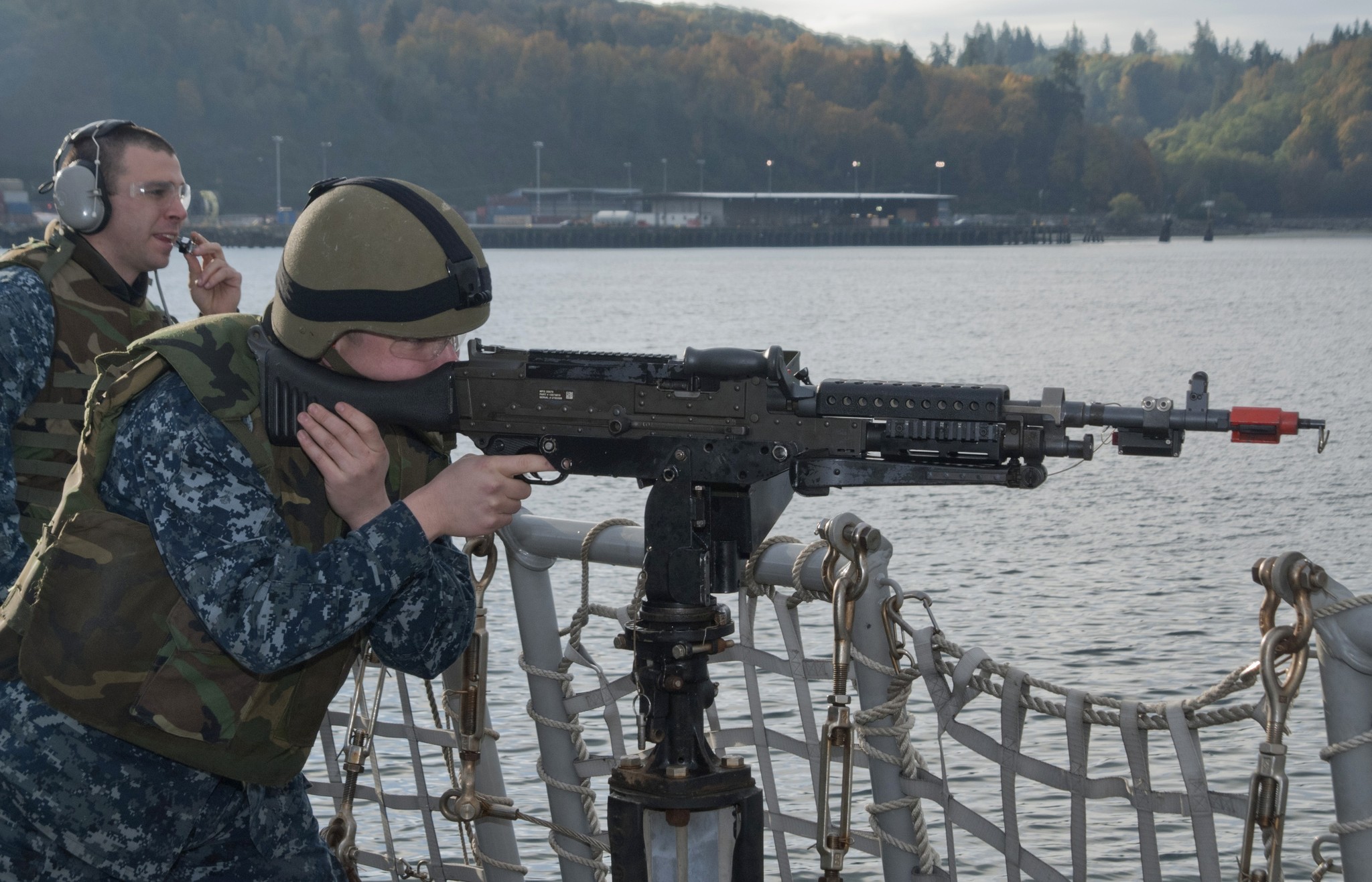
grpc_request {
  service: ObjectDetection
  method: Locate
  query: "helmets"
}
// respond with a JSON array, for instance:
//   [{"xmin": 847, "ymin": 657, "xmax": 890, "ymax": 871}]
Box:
[{"xmin": 270, "ymin": 176, "xmax": 492, "ymax": 360}]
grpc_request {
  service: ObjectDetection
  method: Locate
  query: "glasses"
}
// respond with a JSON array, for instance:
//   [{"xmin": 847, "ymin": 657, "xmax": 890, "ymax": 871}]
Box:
[
  {"xmin": 389, "ymin": 333, "xmax": 468, "ymax": 362},
  {"xmin": 110, "ymin": 180, "xmax": 192, "ymax": 211}
]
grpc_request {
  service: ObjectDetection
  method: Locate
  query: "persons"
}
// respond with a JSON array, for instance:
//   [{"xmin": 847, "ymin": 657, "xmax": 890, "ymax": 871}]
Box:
[
  {"xmin": 0, "ymin": 121, "xmax": 243, "ymax": 619},
  {"xmin": 0, "ymin": 177, "xmax": 555, "ymax": 882}
]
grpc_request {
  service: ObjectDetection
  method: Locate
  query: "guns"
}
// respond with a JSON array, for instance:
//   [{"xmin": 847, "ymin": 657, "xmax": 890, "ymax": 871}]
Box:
[{"xmin": 262, "ymin": 338, "xmax": 1332, "ymax": 764}]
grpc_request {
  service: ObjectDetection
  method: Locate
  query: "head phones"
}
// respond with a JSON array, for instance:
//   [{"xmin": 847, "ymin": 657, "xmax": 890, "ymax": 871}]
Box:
[{"xmin": 52, "ymin": 119, "xmax": 190, "ymax": 236}]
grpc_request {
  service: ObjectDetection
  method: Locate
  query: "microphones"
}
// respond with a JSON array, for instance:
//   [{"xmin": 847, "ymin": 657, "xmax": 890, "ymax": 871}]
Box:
[{"xmin": 174, "ymin": 236, "xmax": 193, "ymax": 254}]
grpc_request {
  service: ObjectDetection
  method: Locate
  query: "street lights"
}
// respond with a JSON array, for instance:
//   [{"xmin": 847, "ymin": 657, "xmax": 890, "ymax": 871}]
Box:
[
  {"xmin": 766, "ymin": 159, "xmax": 772, "ymax": 194},
  {"xmin": 623, "ymin": 162, "xmax": 632, "ymax": 189},
  {"xmin": 852, "ymin": 161, "xmax": 860, "ymax": 192},
  {"xmin": 697, "ymin": 159, "xmax": 705, "ymax": 191},
  {"xmin": 661, "ymin": 159, "xmax": 668, "ymax": 192},
  {"xmin": 534, "ymin": 140, "xmax": 543, "ymax": 224},
  {"xmin": 273, "ymin": 135, "xmax": 283, "ymax": 208},
  {"xmin": 321, "ymin": 140, "xmax": 332, "ymax": 180},
  {"xmin": 936, "ymin": 162, "xmax": 945, "ymax": 194}
]
